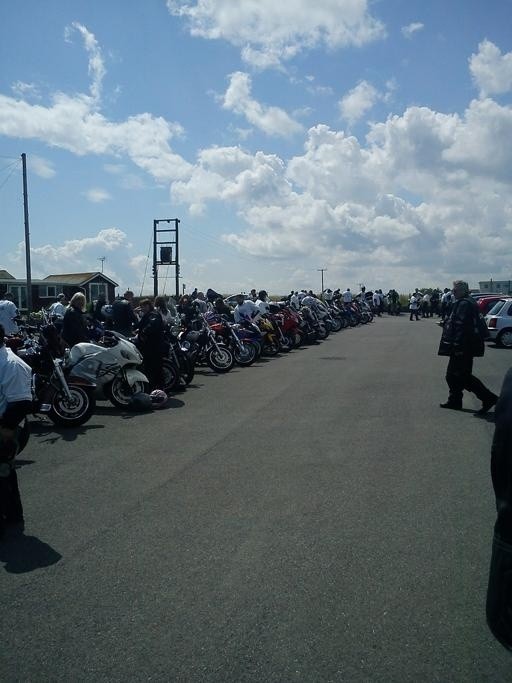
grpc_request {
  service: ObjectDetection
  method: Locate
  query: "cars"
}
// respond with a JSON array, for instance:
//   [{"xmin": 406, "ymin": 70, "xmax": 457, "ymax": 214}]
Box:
[
  {"xmin": 223, "ymin": 292, "xmax": 271, "ymax": 305},
  {"xmin": 470, "ymin": 293, "xmax": 512, "ymax": 349}
]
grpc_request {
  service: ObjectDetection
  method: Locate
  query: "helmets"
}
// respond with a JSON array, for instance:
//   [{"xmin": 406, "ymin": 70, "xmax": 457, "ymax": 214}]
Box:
[
  {"xmin": 148, "ymin": 390, "xmax": 168, "ymax": 410},
  {"xmin": 87, "ymin": 321, "xmax": 104, "ymax": 338},
  {"xmin": 130, "ymin": 392, "xmax": 152, "ymax": 412},
  {"xmin": 204, "ymin": 311, "xmax": 215, "ymax": 321}
]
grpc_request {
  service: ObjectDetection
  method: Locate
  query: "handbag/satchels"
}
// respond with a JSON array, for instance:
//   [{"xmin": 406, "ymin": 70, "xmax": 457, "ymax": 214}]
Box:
[{"xmin": 480, "ymin": 316, "xmax": 490, "ymax": 338}]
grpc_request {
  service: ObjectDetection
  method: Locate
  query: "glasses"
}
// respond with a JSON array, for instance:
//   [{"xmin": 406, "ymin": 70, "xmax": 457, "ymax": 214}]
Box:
[{"xmin": 451, "ymin": 289, "xmax": 457, "ymax": 293}]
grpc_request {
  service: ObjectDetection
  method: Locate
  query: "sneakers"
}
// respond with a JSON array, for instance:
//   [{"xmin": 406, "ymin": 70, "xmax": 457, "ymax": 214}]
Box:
[
  {"xmin": 440, "ymin": 400, "xmax": 462, "ymax": 408},
  {"xmin": 478, "ymin": 395, "xmax": 498, "ymax": 414},
  {"xmin": 0, "ymin": 508, "xmax": 23, "ymax": 522}
]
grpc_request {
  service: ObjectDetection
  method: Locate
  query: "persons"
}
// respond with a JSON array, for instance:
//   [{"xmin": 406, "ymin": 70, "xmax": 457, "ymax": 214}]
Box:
[
  {"xmin": 357, "ymin": 287, "xmax": 401, "ymax": 316},
  {"xmin": 484, "ymin": 365, "xmax": 512, "ymax": 637},
  {"xmin": 437, "ymin": 281, "xmax": 499, "ymax": 414},
  {"xmin": 0, "ymin": 300, "xmax": 31, "ymax": 541},
  {"xmin": 49, "ymin": 287, "xmax": 352, "ymax": 392},
  {"xmin": 409, "ymin": 288, "xmax": 456, "ymax": 320}
]
